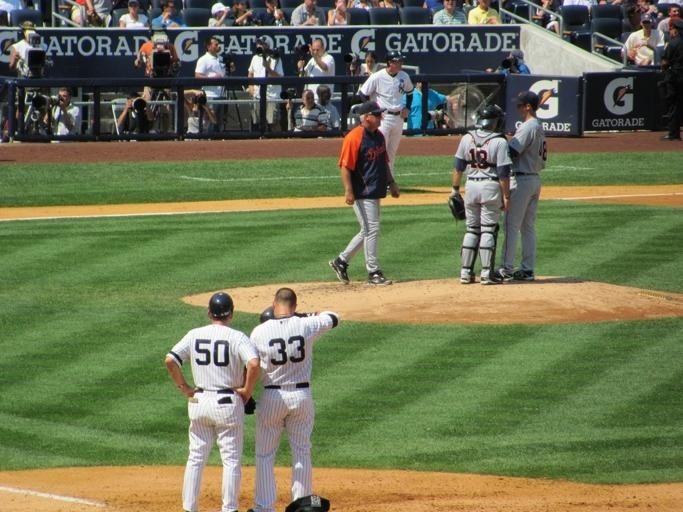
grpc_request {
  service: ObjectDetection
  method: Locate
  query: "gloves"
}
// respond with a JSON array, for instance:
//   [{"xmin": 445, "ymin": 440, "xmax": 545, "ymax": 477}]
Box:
[{"xmin": 245, "ymin": 396, "xmax": 256, "ymax": 414}]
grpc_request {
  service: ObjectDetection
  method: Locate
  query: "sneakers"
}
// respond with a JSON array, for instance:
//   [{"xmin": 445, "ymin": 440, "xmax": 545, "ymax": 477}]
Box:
[
  {"xmin": 328, "ymin": 257, "xmax": 350, "ymax": 285},
  {"xmin": 368, "ymin": 270, "xmax": 392, "ymax": 285},
  {"xmin": 460, "ymin": 269, "xmax": 534, "ymax": 285},
  {"xmin": 659, "ymin": 133, "xmax": 680, "ymax": 141}
]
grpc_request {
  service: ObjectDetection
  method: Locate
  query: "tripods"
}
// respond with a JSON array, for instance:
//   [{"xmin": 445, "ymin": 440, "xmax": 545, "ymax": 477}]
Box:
[
  {"xmin": 148, "ymin": 86, "xmax": 178, "ymax": 135},
  {"xmin": 217, "ymin": 65, "xmax": 243, "ymax": 132}
]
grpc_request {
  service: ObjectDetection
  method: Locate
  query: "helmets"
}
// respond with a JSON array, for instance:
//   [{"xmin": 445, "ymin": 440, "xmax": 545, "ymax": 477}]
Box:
[
  {"xmin": 208, "ymin": 291, "xmax": 233, "ymax": 320},
  {"xmin": 386, "ymin": 50, "xmax": 406, "ymax": 67},
  {"xmin": 477, "ymin": 104, "xmax": 505, "ymax": 127},
  {"xmin": 259, "ymin": 306, "xmax": 274, "ymax": 323}
]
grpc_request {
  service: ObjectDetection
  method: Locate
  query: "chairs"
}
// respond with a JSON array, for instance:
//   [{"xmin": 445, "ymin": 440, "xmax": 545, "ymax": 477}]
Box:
[
  {"xmin": 346, "ymin": 8, "xmax": 370, "ymax": 24},
  {"xmin": 10, "ymin": 10, "xmax": 43, "ymax": 27},
  {"xmin": 400, "ymin": 7, "xmax": 430, "ymax": 24},
  {"xmin": 0, "ymin": 10, "xmax": 9, "ymax": 26},
  {"xmin": 112, "ymin": 8, "xmax": 145, "ymax": 27},
  {"xmin": 178, "ymin": 8, "xmax": 210, "ymax": 26},
  {"xmin": 430, "ymin": 0, "xmax": 683, "ymax": 69},
  {"xmin": 368, "ymin": 8, "xmax": 398, "ymax": 24},
  {"xmin": 221, "ymin": 9, "xmax": 331, "ymax": 26},
  {"xmin": 146, "ymin": 8, "xmax": 162, "ymax": 24}
]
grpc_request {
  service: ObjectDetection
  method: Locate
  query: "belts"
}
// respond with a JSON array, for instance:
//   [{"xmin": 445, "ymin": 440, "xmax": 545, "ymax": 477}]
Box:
[
  {"xmin": 194, "ymin": 388, "xmax": 234, "ymax": 393},
  {"xmin": 469, "ymin": 177, "xmax": 499, "ymax": 181},
  {"xmin": 510, "ymin": 172, "xmax": 538, "ymax": 176},
  {"xmin": 265, "ymin": 383, "xmax": 309, "ymax": 389}
]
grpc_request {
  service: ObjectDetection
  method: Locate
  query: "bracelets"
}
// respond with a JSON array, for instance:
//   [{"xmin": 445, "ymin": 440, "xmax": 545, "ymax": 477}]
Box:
[{"xmin": 387, "ymin": 180, "xmax": 395, "ymax": 184}]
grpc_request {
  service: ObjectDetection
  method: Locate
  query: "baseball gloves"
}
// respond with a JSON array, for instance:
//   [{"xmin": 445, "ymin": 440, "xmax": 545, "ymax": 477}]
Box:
[{"xmin": 447, "ymin": 194, "xmax": 465, "ymax": 220}]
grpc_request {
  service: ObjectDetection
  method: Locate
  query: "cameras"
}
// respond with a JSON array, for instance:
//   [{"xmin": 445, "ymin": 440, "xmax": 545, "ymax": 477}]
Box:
[
  {"xmin": 192, "ymin": 89, "xmax": 207, "ymax": 106},
  {"xmin": 291, "ymin": 43, "xmax": 310, "ymax": 61},
  {"xmin": 501, "ymin": 57, "xmax": 519, "ymax": 73},
  {"xmin": 343, "ymin": 52, "xmax": 360, "ymax": 71},
  {"xmin": 217, "ymin": 50, "xmax": 239, "ymax": 65},
  {"xmin": 279, "ymin": 87, "xmax": 301, "ymax": 100},
  {"xmin": 254, "ymin": 46, "xmax": 285, "ymax": 60}
]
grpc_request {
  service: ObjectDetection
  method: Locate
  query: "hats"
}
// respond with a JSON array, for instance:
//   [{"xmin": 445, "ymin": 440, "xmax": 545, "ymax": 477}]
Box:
[
  {"xmin": 359, "ymin": 101, "xmax": 387, "ymax": 115},
  {"xmin": 511, "ymin": 49, "xmax": 524, "ymax": 59},
  {"xmin": 211, "ymin": 2, "xmax": 225, "ymax": 15},
  {"xmin": 641, "ymin": 14, "xmax": 652, "ymax": 22},
  {"xmin": 509, "ymin": 90, "xmax": 539, "ymax": 106},
  {"xmin": 668, "ymin": 19, "xmax": 683, "ymax": 29},
  {"xmin": 18, "ymin": 20, "xmax": 37, "ymax": 30}
]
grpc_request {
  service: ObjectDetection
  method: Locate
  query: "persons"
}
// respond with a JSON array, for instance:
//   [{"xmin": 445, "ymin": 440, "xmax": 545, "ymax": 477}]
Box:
[
  {"xmin": 496, "ymin": 92, "xmax": 549, "ymax": 282},
  {"xmin": 449, "ymin": 109, "xmax": 513, "ymax": 288},
  {"xmin": 1, "ymin": 1, "xmax": 683, "ymax": 140},
  {"xmin": 246, "ymin": 287, "xmax": 339, "ymax": 512},
  {"xmin": 361, "ymin": 51, "xmax": 416, "ymax": 177},
  {"xmin": 327, "ymin": 102, "xmax": 401, "ymax": 287},
  {"xmin": 163, "ymin": 292, "xmax": 258, "ymax": 512}
]
